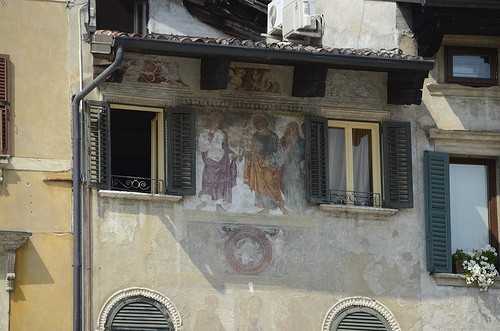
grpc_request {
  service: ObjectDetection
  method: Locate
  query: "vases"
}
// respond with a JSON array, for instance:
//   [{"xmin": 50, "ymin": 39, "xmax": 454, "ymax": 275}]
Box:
[{"xmin": 452, "ymin": 255, "xmax": 471, "ymax": 274}]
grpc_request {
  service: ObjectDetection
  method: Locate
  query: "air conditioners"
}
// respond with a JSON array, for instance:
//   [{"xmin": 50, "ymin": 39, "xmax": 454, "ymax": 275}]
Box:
[
  {"xmin": 267, "ymin": 0, "xmax": 283, "ymax": 35},
  {"xmin": 281, "ymin": 0, "xmax": 317, "ymax": 41}
]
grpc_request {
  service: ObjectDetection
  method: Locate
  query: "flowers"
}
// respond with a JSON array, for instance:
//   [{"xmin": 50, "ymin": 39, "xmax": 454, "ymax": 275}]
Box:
[{"xmin": 456, "ymin": 245, "xmax": 499, "ymax": 292}]
